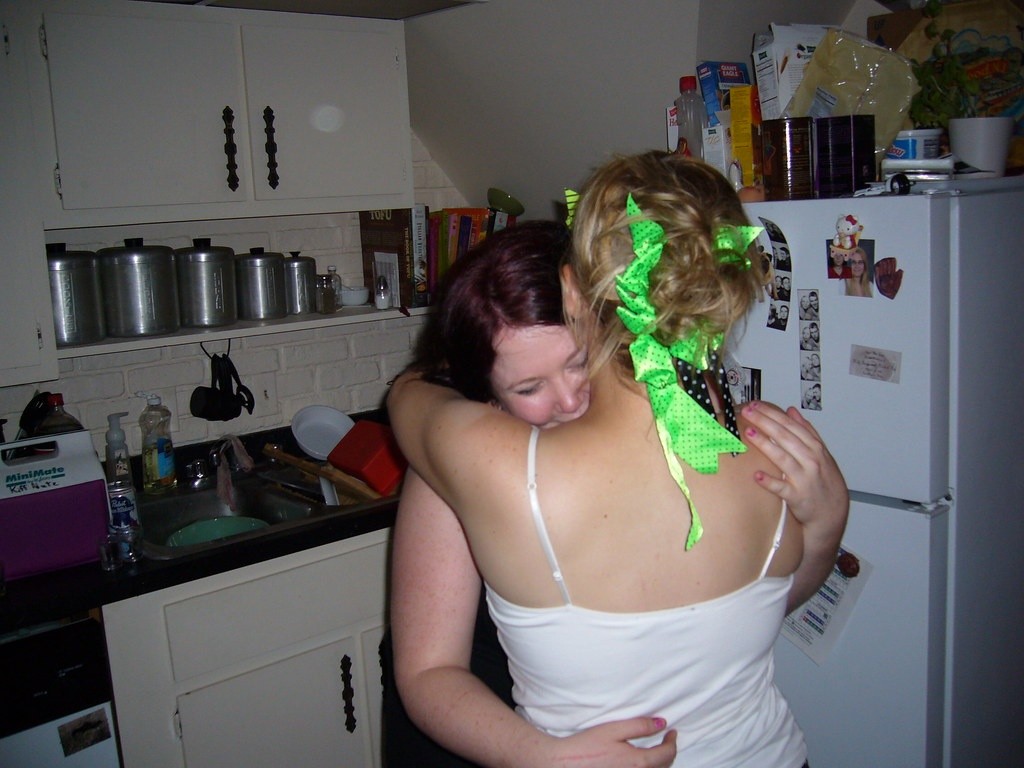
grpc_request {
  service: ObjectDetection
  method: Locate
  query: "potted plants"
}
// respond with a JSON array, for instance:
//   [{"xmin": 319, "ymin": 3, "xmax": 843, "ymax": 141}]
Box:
[{"xmin": 908, "ymin": 0, "xmax": 1023, "ymax": 179}]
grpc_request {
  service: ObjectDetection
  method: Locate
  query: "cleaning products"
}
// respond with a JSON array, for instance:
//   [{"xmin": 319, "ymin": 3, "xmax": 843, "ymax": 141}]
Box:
[
  {"xmin": 104, "ymin": 411, "xmax": 132, "ymax": 484},
  {"xmin": 134, "ymin": 389, "xmax": 179, "ymax": 495}
]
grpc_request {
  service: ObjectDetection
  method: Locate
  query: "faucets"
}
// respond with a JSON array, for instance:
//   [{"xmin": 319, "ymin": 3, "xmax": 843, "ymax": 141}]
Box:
[{"xmin": 208, "ymin": 435, "xmax": 245, "ymax": 481}]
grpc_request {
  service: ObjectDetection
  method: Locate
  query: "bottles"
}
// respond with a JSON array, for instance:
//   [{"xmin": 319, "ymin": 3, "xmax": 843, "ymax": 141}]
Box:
[
  {"xmin": 137, "ymin": 394, "xmax": 177, "ymax": 494},
  {"xmin": 315, "ymin": 274, "xmax": 338, "ymax": 314},
  {"xmin": 327, "ymin": 265, "xmax": 343, "ymax": 309},
  {"xmin": 673, "ymin": 75, "xmax": 705, "ymax": 162},
  {"xmin": 374, "ymin": 276, "xmax": 391, "ymax": 311}
]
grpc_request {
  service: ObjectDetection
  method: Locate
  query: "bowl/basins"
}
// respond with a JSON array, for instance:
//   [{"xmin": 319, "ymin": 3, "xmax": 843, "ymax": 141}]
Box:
[
  {"xmin": 291, "ymin": 405, "xmax": 355, "ymax": 460},
  {"xmin": 169, "ymin": 514, "xmax": 269, "ymax": 548},
  {"xmin": 336, "ymin": 286, "xmax": 369, "ymax": 306}
]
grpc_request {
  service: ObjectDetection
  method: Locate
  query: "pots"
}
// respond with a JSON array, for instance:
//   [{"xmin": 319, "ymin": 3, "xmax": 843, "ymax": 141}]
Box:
[{"xmin": 47, "ymin": 233, "xmax": 316, "ymax": 347}]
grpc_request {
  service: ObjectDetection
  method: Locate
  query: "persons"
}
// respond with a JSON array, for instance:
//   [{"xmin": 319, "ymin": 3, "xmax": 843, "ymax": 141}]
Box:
[
  {"xmin": 386, "ymin": 150, "xmax": 804, "ymax": 768},
  {"xmin": 771, "ymin": 276, "xmax": 791, "ymax": 302},
  {"xmin": 801, "ymin": 383, "xmax": 821, "ymax": 410},
  {"xmin": 799, "ymin": 292, "xmax": 819, "ymax": 321},
  {"xmin": 765, "ymin": 222, "xmax": 787, "ymax": 244},
  {"xmin": 772, "ymin": 246, "xmax": 792, "ymax": 272},
  {"xmin": 766, "ymin": 304, "xmax": 789, "ymax": 331},
  {"xmin": 800, "ymin": 353, "xmax": 820, "ymax": 381},
  {"xmin": 379, "ymin": 220, "xmax": 848, "ymax": 768},
  {"xmin": 800, "ymin": 322, "xmax": 820, "ymax": 351},
  {"xmin": 828, "ymin": 254, "xmax": 852, "ymax": 278},
  {"xmin": 844, "ymin": 247, "xmax": 873, "ymax": 297}
]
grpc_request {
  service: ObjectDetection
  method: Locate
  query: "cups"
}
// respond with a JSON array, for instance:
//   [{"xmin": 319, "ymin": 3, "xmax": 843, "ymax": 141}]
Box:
[
  {"xmin": 886, "ymin": 127, "xmax": 942, "ymax": 159},
  {"xmin": 948, "ymin": 117, "xmax": 1014, "ymax": 176}
]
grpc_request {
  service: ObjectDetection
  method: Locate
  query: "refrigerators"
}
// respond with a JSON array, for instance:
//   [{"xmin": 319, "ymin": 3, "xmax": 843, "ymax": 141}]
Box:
[{"xmin": 693, "ymin": 188, "xmax": 1024, "ymax": 768}]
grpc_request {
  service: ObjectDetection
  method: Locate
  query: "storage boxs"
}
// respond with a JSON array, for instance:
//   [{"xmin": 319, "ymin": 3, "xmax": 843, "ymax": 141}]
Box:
[
  {"xmin": 730, "ymin": 85, "xmax": 763, "ymax": 189},
  {"xmin": 701, "ymin": 111, "xmax": 731, "ymax": 184},
  {"xmin": 751, "ymin": 21, "xmax": 841, "ymax": 120},
  {"xmin": 667, "ymin": 107, "xmax": 680, "ymax": 153},
  {"xmin": 696, "ymin": 59, "xmax": 751, "ymax": 126},
  {"xmin": 328, "ymin": 419, "xmax": 409, "ymax": 497}
]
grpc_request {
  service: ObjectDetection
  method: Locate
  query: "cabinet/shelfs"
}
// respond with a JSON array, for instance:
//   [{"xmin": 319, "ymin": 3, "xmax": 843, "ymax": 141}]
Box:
[
  {"xmin": 39, "ymin": 0, "xmax": 414, "ymax": 230},
  {"xmin": 163, "ymin": 539, "xmax": 476, "ymax": 768}
]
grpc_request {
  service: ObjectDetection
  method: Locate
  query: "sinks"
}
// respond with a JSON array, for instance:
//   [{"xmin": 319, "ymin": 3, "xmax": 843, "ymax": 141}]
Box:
[{"xmin": 128, "ymin": 471, "xmax": 316, "ymax": 561}]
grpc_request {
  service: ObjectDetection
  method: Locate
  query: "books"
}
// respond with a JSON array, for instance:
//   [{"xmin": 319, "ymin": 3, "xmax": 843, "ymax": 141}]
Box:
[{"xmin": 359, "ymin": 204, "xmax": 515, "ymax": 307}]
